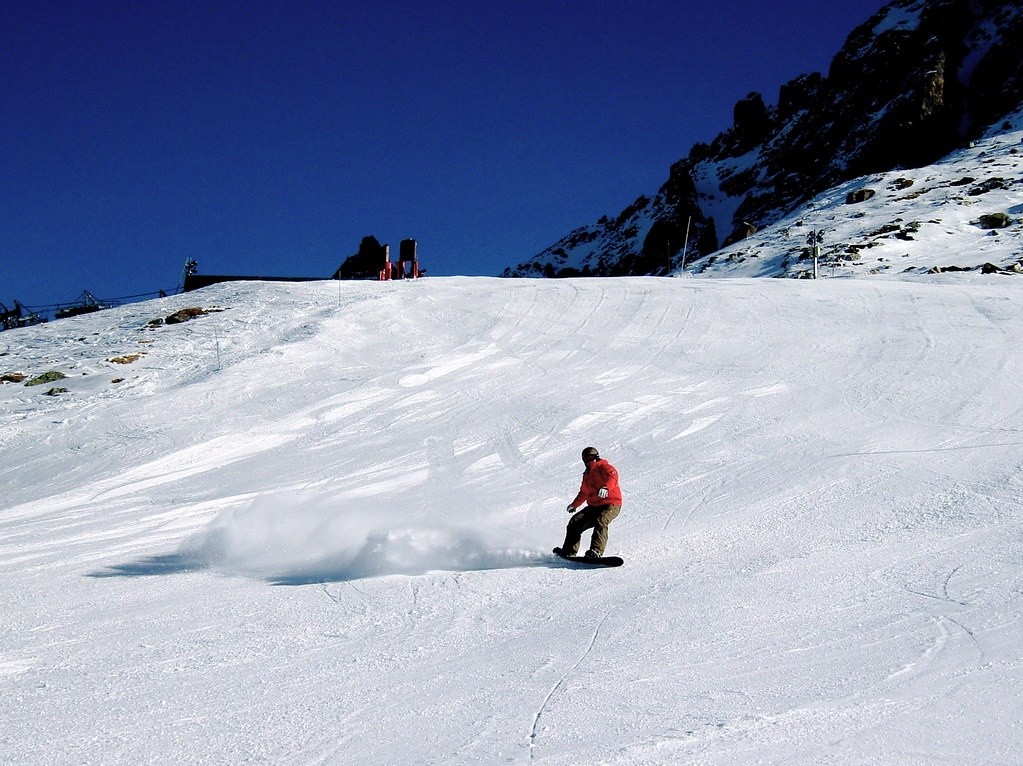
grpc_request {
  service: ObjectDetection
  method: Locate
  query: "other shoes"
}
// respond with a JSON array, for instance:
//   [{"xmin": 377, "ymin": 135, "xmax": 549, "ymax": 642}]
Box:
[
  {"xmin": 585, "ymin": 549, "xmax": 601, "ymax": 558},
  {"xmin": 557, "ymin": 547, "xmax": 576, "ymax": 556}
]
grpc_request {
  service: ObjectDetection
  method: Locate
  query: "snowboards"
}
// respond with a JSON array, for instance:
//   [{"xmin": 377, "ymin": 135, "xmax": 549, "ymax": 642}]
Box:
[{"xmin": 552, "ymin": 549, "xmax": 624, "ymax": 567}]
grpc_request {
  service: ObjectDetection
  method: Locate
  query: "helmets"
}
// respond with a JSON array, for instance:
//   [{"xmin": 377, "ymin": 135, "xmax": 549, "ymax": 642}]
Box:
[{"xmin": 582, "ymin": 447, "xmax": 600, "ymax": 463}]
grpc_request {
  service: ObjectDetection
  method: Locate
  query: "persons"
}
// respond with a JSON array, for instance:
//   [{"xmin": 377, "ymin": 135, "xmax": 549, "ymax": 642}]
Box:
[{"xmin": 552, "ymin": 447, "xmax": 622, "ymax": 558}]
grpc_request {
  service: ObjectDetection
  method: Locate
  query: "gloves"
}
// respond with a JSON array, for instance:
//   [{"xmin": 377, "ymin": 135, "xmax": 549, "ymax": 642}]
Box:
[
  {"xmin": 567, "ymin": 505, "xmax": 576, "ymax": 513},
  {"xmin": 598, "ymin": 487, "xmax": 609, "ymax": 499}
]
